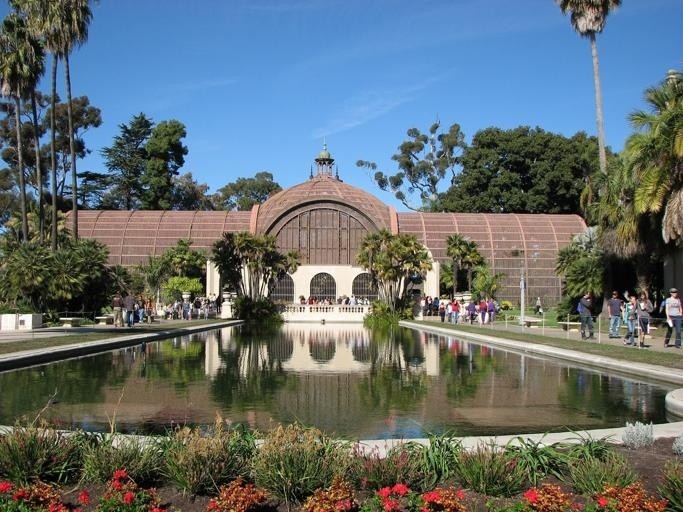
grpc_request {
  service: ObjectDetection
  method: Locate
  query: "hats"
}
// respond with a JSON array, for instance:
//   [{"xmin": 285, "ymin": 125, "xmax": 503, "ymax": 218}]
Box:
[{"xmin": 670, "ymin": 288, "xmax": 679, "ymax": 293}]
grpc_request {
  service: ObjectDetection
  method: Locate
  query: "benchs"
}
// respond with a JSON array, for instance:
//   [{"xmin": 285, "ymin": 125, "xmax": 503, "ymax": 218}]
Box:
[
  {"xmin": 59, "ymin": 317, "xmax": 84, "ymax": 327},
  {"xmin": 621, "ymin": 325, "xmax": 659, "ymax": 339},
  {"xmin": 558, "ymin": 322, "xmax": 582, "ymax": 332},
  {"xmin": 523, "ymin": 318, "xmax": 543, "ymax": 329},
  {"xmin": 95, "ymin": 316, "xmax": 112, "ymax": 325}
]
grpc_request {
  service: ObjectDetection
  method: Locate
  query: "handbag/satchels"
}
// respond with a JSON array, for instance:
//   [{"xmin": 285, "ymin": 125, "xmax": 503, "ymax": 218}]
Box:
[{"xmin": 577, "ymin": 303, "xmax": 583, "ymax": 313}]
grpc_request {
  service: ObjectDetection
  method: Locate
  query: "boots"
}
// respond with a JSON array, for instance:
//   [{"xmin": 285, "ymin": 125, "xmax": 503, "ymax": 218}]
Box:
[{"xmin": 581, "ymin": 332, "xmax": 597, "ymax": 340}]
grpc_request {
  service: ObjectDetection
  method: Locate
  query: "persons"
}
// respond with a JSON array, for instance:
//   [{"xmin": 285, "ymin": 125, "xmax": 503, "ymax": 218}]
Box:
[
  {"xmin": 124, "ymin": 291, "xmax": 135, "ymax": 328},
  {"xmin": 605, "ymin": 291, "xmax": 622, "ymax": 338},
  {"xmin": 621, "ymin": 294, "xmax": 636, "ymax": 346},
  {"xmin": 661, "ymin": 287, "xmax": 683, "ymax": 348},
  {"xmin": 298, "ymin": 295, "xmax": 371, "ymax": 312},
  {"xmin": 534, "ymin": 296, "xmax": 542, "ymax": 315},
  {"xmin": 413, "ymin": 292, "xmax": 495, "ymax": 325},
  {"xmin": 112, "ymin": 292, "xmax": 123, "ymax": 327},
  {"xmin": 136, "ymin": 295, "xmax": 221, "ymax": 325},
  {"xmin": 632, "ymin": 291, "xmax": 653, "ymax": 348},
  {"xmin": 577, "ymin": 290, "xmax": 595, "ymax": 341}
]
